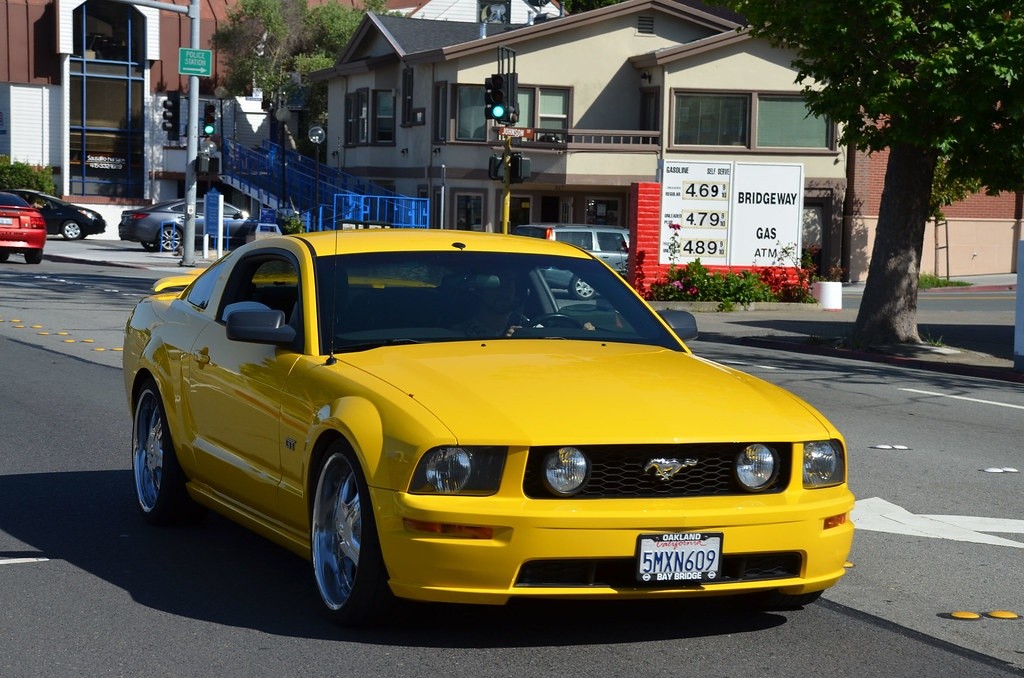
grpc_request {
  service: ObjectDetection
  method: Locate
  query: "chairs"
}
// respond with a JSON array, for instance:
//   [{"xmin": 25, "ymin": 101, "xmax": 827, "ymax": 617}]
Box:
[
  {"xmin": 287, "ymin": 267, "xmax": 358, "ymax": 343},
  {"xmin": 434, "ymin": 270, "xmax": 499, "ymax": 328}
]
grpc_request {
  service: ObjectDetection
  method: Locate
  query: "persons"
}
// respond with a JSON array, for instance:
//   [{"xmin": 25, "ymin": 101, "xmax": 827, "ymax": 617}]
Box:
[
  {"xmin": 450, "ymin": 271, "xmax": 597, "ymax": 337},
  {"xmin": 27, "ymin": 195, "xmax": 46, "ymax": 207}
]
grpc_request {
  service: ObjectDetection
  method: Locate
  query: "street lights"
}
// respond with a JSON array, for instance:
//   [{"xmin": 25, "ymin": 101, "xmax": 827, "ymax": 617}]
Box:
[{"xmin": 274, "ymin": 106, "xmax": 292, "ymax": 206}]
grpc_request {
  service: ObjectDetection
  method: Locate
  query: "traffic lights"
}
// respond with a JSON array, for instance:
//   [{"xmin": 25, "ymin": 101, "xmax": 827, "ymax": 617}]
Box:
[
  {"xmin": 490, "ymin": 74, "xmax": 511, "ymax": 124},
  {"xmin": 483, "ymin": 77, "xmax": 493, "ymax": 119},
  {"xmin": 204, "ymin": 101, "xmax": 217, "ymax": 136},
  {"xmin": 160, "ymin": 88, "xmax": 181, "ymax": 140},
  {"xmin": 511, "ymin": 154, "xmax": 532, "ymax": 180}
]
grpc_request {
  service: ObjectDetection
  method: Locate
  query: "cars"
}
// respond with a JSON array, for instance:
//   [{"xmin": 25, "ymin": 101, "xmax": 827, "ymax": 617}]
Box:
[
  {"xmin": 0, "ymin": 191, "xmax": 47, "ymax": 264},
  {"xmin": 0, "ymin": 188, "xmax": 107, "ymax": 241},
  {"xmin": 118, "ymin": 198, "xmax": 259, "ymax": 251},
  {"xmin": 121, "ymin": 228, "xmax": 858, "ymax": 629}
]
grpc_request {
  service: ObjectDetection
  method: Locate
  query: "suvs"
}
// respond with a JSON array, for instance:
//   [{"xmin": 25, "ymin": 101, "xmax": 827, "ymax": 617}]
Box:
[{"xmin": 513, "ymin": 227, "xmax": 632, "ymax": 299}]
